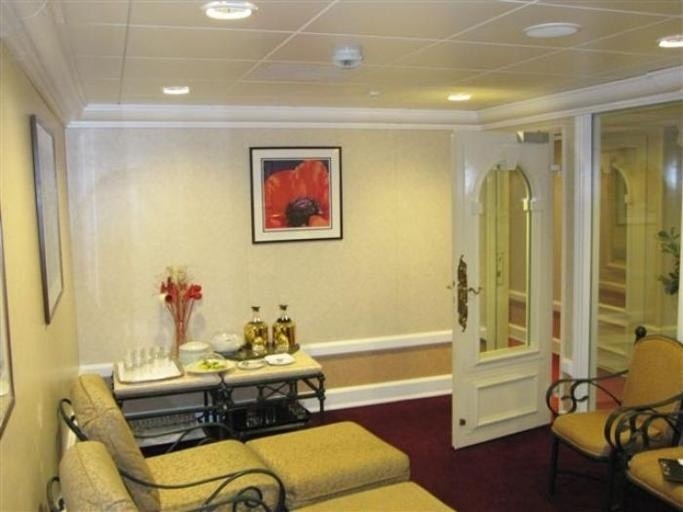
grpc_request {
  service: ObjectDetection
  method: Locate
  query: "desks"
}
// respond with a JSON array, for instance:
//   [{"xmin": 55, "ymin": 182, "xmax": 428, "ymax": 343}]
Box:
[
  {"xmin": 112, "ymin": 364, "xmax": 224, "ymax": 455},
  {"xmin": 223, "ymin": 349, "xmax": 326, "ymax": 439}
]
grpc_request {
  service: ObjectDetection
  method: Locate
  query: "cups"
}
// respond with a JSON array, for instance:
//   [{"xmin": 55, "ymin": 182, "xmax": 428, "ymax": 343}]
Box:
[{"xmin": 122, "ymin": 344, "xmax": 166, "ymax": 376}]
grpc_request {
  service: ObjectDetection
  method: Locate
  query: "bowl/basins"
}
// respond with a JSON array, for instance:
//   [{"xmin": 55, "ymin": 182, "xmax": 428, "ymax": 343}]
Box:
[{"xmin": 211, "ymin": 333, "xmax": 248, "ymax": 357}]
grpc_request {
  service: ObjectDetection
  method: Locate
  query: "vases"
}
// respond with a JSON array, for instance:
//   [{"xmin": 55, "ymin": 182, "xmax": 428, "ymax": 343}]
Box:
[{"xmin": 170, "ymin": 332, "xmax": 186, "ymax": 362}]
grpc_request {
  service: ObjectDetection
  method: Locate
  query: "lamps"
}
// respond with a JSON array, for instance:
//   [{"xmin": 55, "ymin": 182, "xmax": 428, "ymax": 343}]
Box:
[
  {"xmin": 656, "ymin": 34, "xmax": 683, "ymax": 49},
  {"xmin": 522, "ymin": 22, "xmax": 581, "ymax": 39},
  {"xmin": 201, "ymin": 0, "xmax": 259, "ymax": 20}
]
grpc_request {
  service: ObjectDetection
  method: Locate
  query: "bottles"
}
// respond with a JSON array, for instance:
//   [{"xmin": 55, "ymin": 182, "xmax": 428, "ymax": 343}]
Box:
[
  {"xmin": 243, "ymin": 306, "xmax": 270, "ymax": 356},
  {"xmin": 272, "ymin": 303, "xmax": 296, "ymax": 354}
]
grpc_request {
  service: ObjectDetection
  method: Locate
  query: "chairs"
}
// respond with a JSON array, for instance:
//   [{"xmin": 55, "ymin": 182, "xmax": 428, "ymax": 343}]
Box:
[
  {"xmin": 546, "ymin": 325, "xmax": 683, "ymax": 512},
  {"xmin": 615, "ymin": 412, "xmax": 683, "ymax": 511},
  {"xmin": 59, "ymin": 373, "xmax": 412, "ymax": 512},
  {"xmin": 46, "ymin": 440, "xmax": 459, "ymax": 512}
]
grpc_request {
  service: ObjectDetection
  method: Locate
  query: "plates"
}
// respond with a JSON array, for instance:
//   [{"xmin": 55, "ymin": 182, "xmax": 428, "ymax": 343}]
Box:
[
  {"xmin": 186, "ymin": 360, "xmax": 236, "ymax": 375},
  {"xmin": 265, "ymin": 352, "xmax": 296, "ymax": 367},
  {"xmin": 238, "ymin": 358, "xmax": 265, "ymax": 370},
  {"xmin": 178, "ymin": 341, "xmax": 211, "ymax": 365}
]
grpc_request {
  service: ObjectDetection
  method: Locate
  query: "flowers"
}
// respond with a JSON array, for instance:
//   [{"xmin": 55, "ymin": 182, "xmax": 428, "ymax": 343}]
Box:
[{"xmin": 152, "ymin": 266, "xmax": 202, "ymax": 353}]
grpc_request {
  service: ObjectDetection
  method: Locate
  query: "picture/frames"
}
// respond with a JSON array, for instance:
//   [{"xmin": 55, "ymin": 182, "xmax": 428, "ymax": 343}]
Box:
[
  {"xmin": 30, "ymin": 113, "xmax": 66, "ymax": 323},
  {"xmin": 249, "ymin": 146, "xmax": 343, "ymax": 244},
  {"xmin": 0, "ymin": 210, "xmax": 17, "ymax": 439}
]
